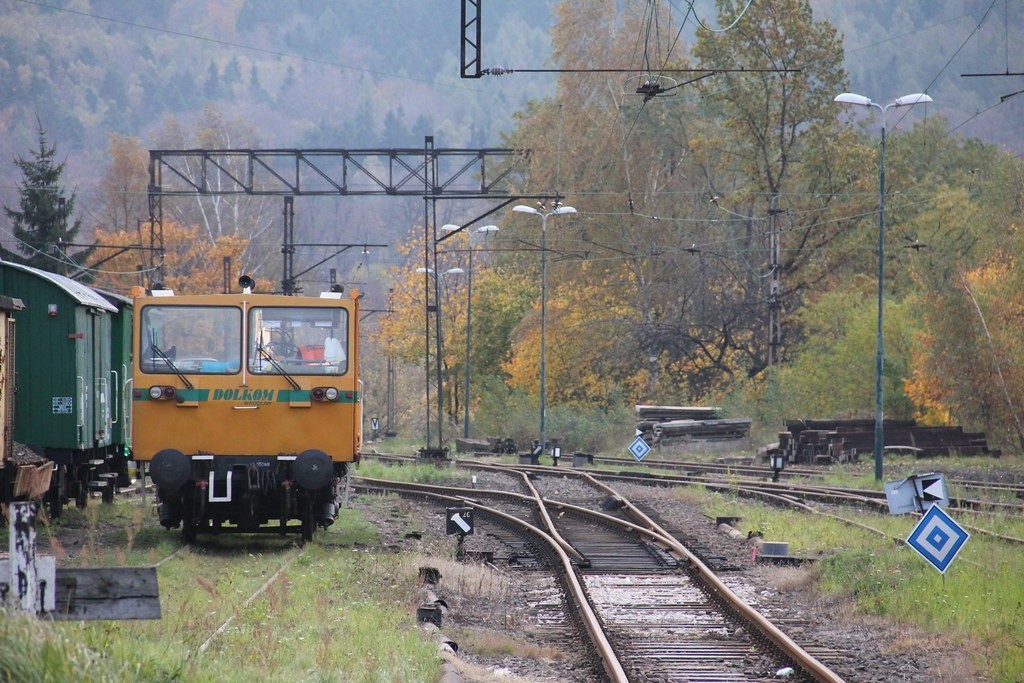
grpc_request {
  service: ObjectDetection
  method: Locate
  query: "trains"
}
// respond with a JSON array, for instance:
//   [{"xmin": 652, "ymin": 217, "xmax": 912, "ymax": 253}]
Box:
[{"xmin": 0, "ymin": 250, "xmax": 366, "ymax": 544}]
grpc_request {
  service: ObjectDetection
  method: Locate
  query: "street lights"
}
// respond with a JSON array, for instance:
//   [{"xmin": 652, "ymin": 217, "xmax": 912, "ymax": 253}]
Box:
[
  {"xmin": 836, "ymin": 90, "xmax": 934, "ymax": 484},
  {"xmin": 416, "ymin": 205, "xmax": 577, "ymax": 456}
]
